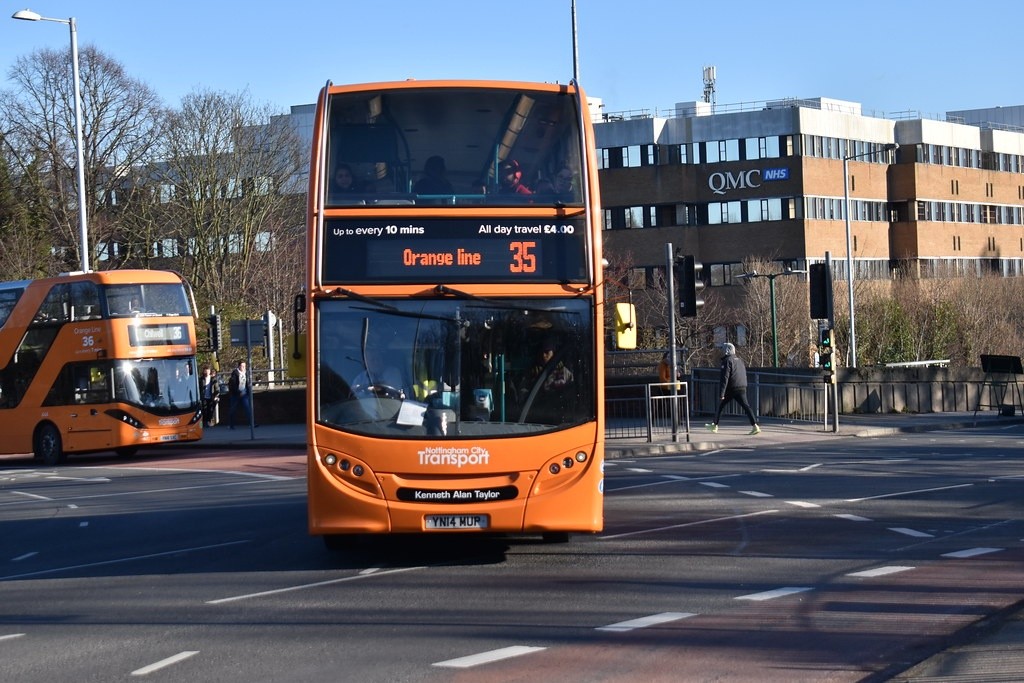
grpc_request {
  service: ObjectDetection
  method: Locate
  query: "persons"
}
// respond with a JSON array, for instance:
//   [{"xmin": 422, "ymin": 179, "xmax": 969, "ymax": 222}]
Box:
[
  {"xmin": 227, "ymin": 359, "xmax": 259, "ymax": 431},
  {"xmin": 703, "ymin": 344, "xmax": 765, "ymax": 436},
  {"xmin": 410, "ymin": 154, "xmax": 455, "ymax": 195},
  {"xmin": 659, "ymin": 351, "xmax": 681, "ymax": 422},
  {"xmin": 164, "ymin": 363, "xmax": 198, "ymax": 402},
  {"xmin": 199, "ymin": 363, "xmax": 224, "ymax": 429},
  {"xmin": 539, "ymin": 167, "xmax": 575, "ymax": 202},
  {"xmin": 524, "ymin": 341, "xmax": 574, "ymax": 406},
  {"xmin": 350, "ymin": 341, "xmax": 404, "ymax": 405},
  {"xmin": 332, "ymin": 166, "xmax": 377, "ymax": 200},
  {"xmin": 497, "ymin": 158, "xmax": 536, "ymax": 204},
  {"xmin": 126, "ymin": 298, "xmax": 145, "ymax": 313}
]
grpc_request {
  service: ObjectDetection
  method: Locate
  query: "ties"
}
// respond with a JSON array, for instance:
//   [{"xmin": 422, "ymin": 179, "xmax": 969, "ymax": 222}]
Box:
[{"xmin": 372, "ymin": 374, "xmax": 380, "ymax": 384}]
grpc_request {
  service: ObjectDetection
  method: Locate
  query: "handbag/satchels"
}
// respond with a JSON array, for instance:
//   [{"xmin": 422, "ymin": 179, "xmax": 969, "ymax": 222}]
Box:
[{"xmin": 667, "ymin": 380, "xmax": 680, "ymax": 390}]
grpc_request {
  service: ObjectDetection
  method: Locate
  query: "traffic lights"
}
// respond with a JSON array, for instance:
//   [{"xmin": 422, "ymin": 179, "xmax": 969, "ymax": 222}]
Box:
[
  {"xmin": 678, "ymin": 255, "xmax": 705, "ymax": 317},
  {"xmin": 822, "ymin": 329, "xmax": 830, "ymax": 370}
]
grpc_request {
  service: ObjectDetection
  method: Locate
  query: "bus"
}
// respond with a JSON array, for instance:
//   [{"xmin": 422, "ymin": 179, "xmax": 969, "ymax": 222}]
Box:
[
  {"xmin": 1, "ymin": 269, "xmax": 219, "ymax": 468},
  {"xmin": 287, "ymin": 77, "xmax": 637, "ymax": 545}
]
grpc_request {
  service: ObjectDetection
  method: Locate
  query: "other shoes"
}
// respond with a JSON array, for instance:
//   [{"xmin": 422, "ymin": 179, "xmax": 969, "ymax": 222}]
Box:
[
  {"xmin": 705, "ymin": 423, "xmax": 718, "ymax": 433},
  {"xmin": 249, "ymin": 422, "xmax": 259, "ymax": 429},
  {"xmin": 749, "ymin": 425, "xmax": 760, "ymax": 435},
  {"xmin": 227, "ymin": 425, "xmax": 234, "ymax": 430}
]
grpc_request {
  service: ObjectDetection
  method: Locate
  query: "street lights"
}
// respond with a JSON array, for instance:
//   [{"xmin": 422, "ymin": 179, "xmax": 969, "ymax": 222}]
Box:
[
  {"xmin": 843, "ymin": 142, "xmax": 899, "ymax": 369},
  {"xmin": 733, "ymin": 267, "xmax": 810, "ymax": 369},
  {"xmin": 13, "ymin": 8, "xmax": 89, "ymax": 273}
]
